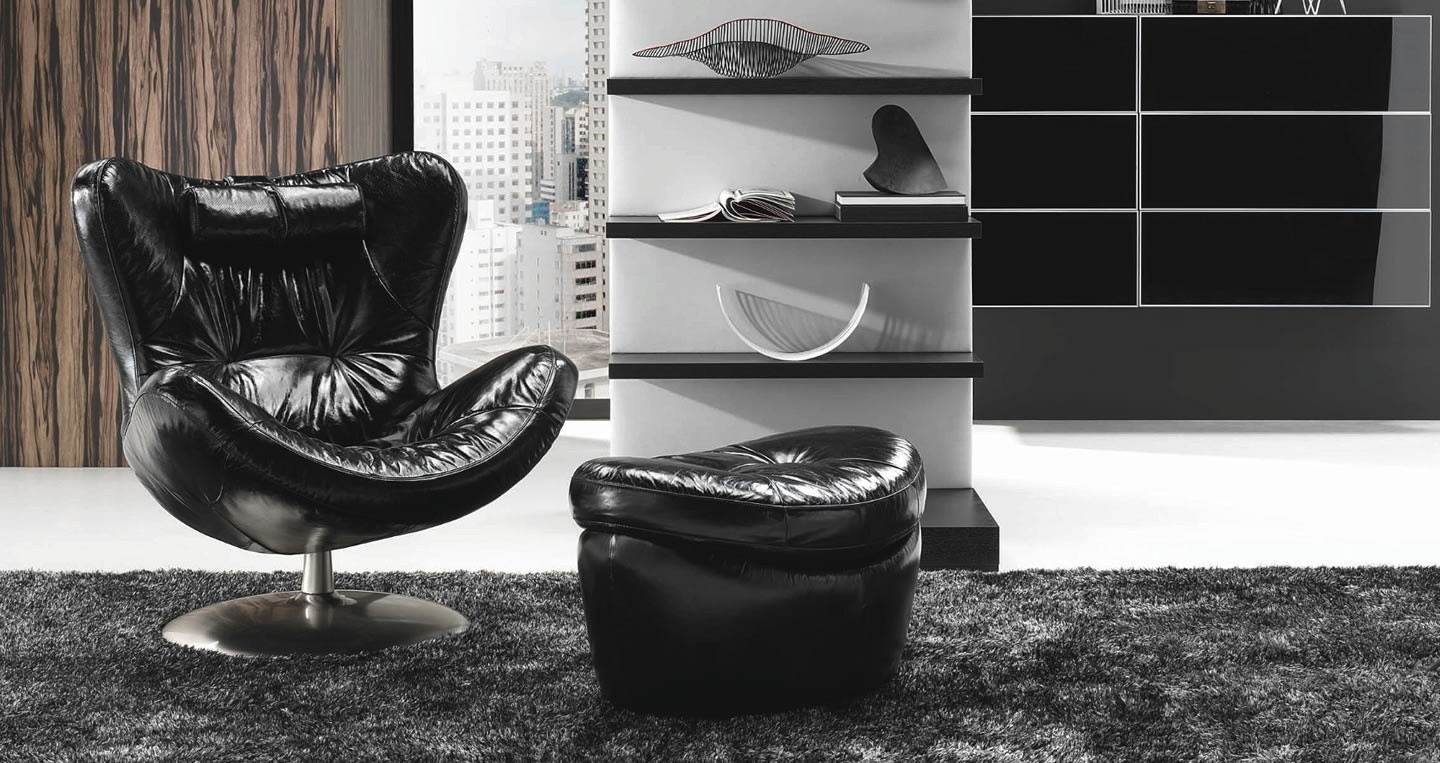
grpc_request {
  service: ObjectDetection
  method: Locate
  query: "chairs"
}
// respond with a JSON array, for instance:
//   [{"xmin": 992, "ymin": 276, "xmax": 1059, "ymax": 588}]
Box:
[{"xmin": 68, "ymin": 148, "xmax": 578, "ymax": 663}]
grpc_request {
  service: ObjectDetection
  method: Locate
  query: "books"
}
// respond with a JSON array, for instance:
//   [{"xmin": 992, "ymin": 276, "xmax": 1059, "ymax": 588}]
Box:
[
  {"xmin": 658, "ymin": 202, "xmax": 779, "ymax": 223},
  {"xmin": 719, "ymin": 187, "xmax": 796, "ymax": 222},
  {"xmin": 835, "ymin": 190, "xmax": 967, "ymax": 204}
]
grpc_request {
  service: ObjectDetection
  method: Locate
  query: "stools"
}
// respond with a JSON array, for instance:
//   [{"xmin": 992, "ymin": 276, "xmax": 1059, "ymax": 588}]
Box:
[{"xmin": 569, "ymin": 423, "xmax": 928, "ymax": 721}]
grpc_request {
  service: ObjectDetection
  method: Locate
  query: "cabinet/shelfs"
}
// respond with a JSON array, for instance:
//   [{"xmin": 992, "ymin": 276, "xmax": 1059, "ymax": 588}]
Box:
[{"xmin": 604, "ymin": 74, "xmax": 1006, "ymax": 576}]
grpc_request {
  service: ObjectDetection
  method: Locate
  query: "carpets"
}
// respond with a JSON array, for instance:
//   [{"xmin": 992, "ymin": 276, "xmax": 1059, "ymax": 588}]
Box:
[{"xmin": 0, "ymin": 567, "xmax": 1440, "ymax": 763}]
